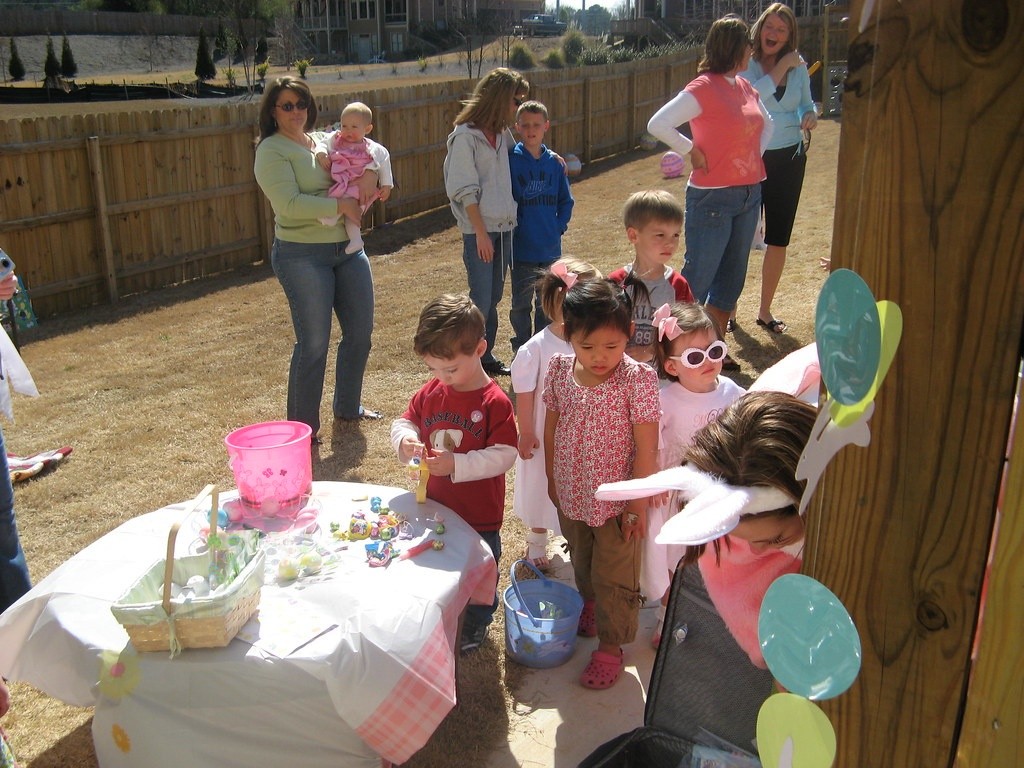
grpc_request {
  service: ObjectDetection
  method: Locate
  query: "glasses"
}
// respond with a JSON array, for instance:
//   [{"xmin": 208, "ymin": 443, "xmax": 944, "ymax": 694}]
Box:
[
  {"xmin": 272, "ymin": 99, "xmax": 309, "ymax": 112},
  {"xmin": 785, "ymin": 125, "xmax": 811, "ymax": 155},
  {"xmin": 665, "ymin": 340, "xmax": 728, "ymax": 369},
  {"xmin": 512, "ymin": 96, "xmax": 523, "ymax": 107}
]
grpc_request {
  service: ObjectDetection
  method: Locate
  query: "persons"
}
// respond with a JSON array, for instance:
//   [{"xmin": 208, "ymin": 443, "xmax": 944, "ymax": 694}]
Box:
[
  {"xmin": 511, "ymin": 261, "xmax": 663, "ymax": 692},
  {"xmin": 0, "ymin": 272, "xmax": 21, "ymax": 768},
  {"xmin": 254, "ymin": 77, "xmax": 381, "ymax": 442},
  {"xmin": 639, "ymin": 300, "xmax": 745, "ymax": 649},
  {"xmin": 727, "ymin": 3, "xmax": 818, "ymax": 333},
  {"xmin": 595, "ymin": 341, "xmax": 821, "ymax": 555},
  {"xmin": 316, "ymin": 101, "xmax": 393, "ymax": 253},
  {"xmin": 443, "ymin": 69, "xmax": 567, "ymax": 372},
  {"xmin": 508, "ymin": 101, "xmax": 573, "ymax": 352},
  {"xmin": 391, "ymin": 295, "xmax": 518, "ymax": 651},
  {"xmin": 646, "ymin": 15, "xmax": 776, "ymax": 371},
  {"xmin": 610, "ymin": 191, "xmax": 695, "ymax": 389}
]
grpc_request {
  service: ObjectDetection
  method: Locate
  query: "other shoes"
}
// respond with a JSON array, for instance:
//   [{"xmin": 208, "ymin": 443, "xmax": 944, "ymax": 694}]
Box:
[
  {"xmin": 311, "ymin": 434, "xmax": 323, "ymax": 446},
  {"xmin": 526, "ymin": 547, "xmax": 549, "ymax": 571},
  {"xmin": 483, "ymin": 361, "xmax": 512, "ymax": 375},
  {"xmin": 360, "ymin": 409, "xmax": 382, "ymax": 419},
  {"xmin": 458, "ymin": 621, "xmax": 489, "ymax": 651},
  {"xmin": 652, "ymin": 619, "xmax": 662, "ymax": 650}
]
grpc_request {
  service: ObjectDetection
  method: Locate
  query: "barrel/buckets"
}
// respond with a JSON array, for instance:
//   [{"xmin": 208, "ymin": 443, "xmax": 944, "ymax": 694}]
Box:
[
  {"xmin": 503, "ymin": 560, "xmax": 584, "ymax": 667},
  {"xmin": 225, "ymin": 421, "xmax": 313, "ymax": 510}
]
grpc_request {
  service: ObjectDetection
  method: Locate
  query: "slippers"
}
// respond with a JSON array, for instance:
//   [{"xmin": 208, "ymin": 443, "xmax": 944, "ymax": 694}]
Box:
[
  {"xmin": 726, "ymin": 318, "xmax": 736, "ymax": 333},
  {"xmin": 577, "ymin": 596, "xmax": 597, "ymax": 637},
  {"xmin": 757, "ymin": 315, "xmax": 788, "ymax": 334},
  {"xmin": 581, "ymin": 647, "xmax": 624, "ymax": 691}
]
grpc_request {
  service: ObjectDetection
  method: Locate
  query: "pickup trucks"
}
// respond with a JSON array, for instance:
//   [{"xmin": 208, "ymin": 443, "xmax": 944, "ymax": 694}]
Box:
[{"xmin": 514, "ymin": 14, "xmax": 569, "ymax": 36}]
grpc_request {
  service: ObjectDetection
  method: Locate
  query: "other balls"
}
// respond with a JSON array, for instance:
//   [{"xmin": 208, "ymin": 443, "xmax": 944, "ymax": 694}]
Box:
[
  {"xmin": 561, "ymin": 154, "xmax": 582, "ymax": 180},
  {"xmin": 640, "ymin": 132, "xmax": 658, "ymax": 151},
  {"xmin": 660, "ymin": 152, "xmax": 685, "ymax": 178}
]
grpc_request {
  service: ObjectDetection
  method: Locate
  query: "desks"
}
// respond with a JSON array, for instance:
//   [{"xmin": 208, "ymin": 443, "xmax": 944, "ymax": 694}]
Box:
[{"xmin": 0, "ymin": 479, "xmax": 496, "ymax": 767}]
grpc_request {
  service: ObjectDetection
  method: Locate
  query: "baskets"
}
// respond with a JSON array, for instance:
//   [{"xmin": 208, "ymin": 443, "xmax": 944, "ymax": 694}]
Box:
[{"xmin": 110, "ymin": 483, "xmax": 266, "ymax": 651}]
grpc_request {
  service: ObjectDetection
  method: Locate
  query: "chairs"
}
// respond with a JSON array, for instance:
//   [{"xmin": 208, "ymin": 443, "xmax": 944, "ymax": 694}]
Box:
[{"xmin": 573, "ymin": 551, "xmax": 781, "ymax": 767}]
